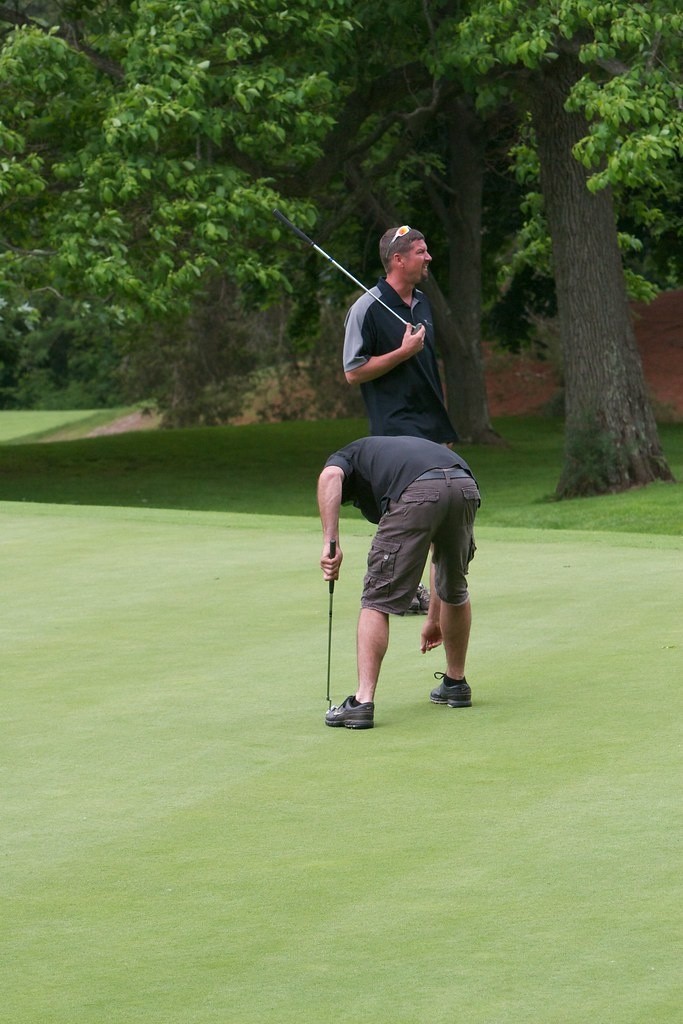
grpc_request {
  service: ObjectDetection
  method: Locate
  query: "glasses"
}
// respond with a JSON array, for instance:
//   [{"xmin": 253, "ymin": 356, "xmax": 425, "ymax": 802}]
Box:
[{"xmin": 386, "ymin": 225, "xmax": 411, "ymax": 259}]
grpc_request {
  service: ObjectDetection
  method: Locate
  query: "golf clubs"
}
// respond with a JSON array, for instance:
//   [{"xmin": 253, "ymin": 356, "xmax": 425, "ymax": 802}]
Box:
[
  {"xmin": 326, "ymin": 539, "xmax": 337, "ymax": 709},
  {"xmin": 272, "ymin": 209, "xmax": 424, "ymax": 334}
]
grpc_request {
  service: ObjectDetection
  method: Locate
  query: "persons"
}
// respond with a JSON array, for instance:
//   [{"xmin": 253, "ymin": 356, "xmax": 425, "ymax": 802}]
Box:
[
  {"xmin": 343, "ymin": 225, "xmax": 461, "ymax": 615},
  {"xmin": 317, "ymin": 436, "xmax": 481, "ymax": 729}
]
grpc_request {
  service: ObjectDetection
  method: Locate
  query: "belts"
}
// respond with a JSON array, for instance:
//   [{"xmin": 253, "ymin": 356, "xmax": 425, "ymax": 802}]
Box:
[{"xmin": 415, "ymin": 470, "xmax": 471, "ymax": 479}]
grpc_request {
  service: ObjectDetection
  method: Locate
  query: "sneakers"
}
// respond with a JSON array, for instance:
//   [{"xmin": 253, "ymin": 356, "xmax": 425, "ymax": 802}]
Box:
[
  {"xmin": 325, "ymin": 695, "xmax": 374, "ymax": 728},
  {"xmin": 407, "ymin": 583, "xmax": 430, "ymax": 614},
  {"xmin": 430, "ymin": 672, "xmax": 472, "ymax": 707}
]
